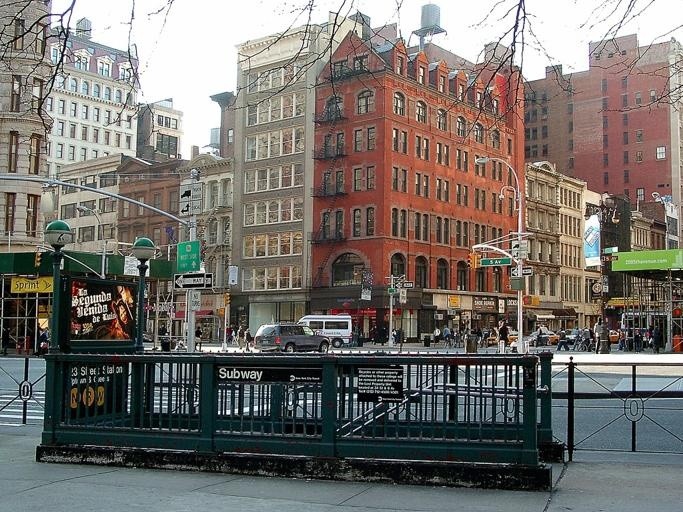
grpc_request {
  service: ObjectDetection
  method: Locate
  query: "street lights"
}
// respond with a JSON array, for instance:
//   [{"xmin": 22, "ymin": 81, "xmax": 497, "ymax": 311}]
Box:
[
  {"xmin": 475, "ymin": 157, "xmax": 523, "ymax": 353},
  {"xmin": 76, "ymin": 206, "xmax": 107, "ymax": 278}
]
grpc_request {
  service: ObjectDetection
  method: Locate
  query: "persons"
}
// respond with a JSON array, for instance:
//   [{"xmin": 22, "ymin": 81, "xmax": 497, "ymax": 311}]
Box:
[
  {"xmin": 369, "ymin": 325, "xmax": 387, "ymax": 346},
  {"xmin": 431, "ymin": 325, "xmax": 465, "ymax": 348},
  {"xmin": 194, "ymin": 326, "xmax": 204, "ymax": 354},
  {"xmin": 616, "ymin": 326, "xmax": 662, "ymax": 355},
  {"xmin": 227, "ymin": 325, "xmax": 253, "ymax": 351},
  {"xmin": 556, "ymin": 317, "xmax": 603, "ymax": 355},
  {"xmin": 392, "ymin": 329, "xmax": 398, "ymax": 345},
  {"xmin": 37, "ymin": 323, "xmax": 49, "ymax": 342},
  {"xmin": 478, "ymin": 319, "xmax": 511, "ymax": 352}
]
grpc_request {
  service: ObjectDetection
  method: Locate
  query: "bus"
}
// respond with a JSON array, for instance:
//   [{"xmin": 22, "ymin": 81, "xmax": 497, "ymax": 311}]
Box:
[{"xmin": 604, "ymin": 297, "xmax": 670, "ymax": 347}]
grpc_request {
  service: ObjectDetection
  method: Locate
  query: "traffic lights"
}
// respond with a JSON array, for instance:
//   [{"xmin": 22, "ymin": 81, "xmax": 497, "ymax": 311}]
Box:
[
  {"xmin": 523, "ymin": 295, "xmax": 540, "ymax": 306},
  {"xmin": 467, "ymin": 253, "xmax": 483, "ymax": 270}
]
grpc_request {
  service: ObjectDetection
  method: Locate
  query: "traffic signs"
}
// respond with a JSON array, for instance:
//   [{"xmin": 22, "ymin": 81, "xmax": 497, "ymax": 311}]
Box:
[
  {"xmin": 394, "ymin": 281, "xmax": 414, "ymax": 289},
  {"xmin": 511, "ymin": 265, "xmax": 534, "ymax": 278},
  {"xmin": 174, "ymin": 273, "xmax": 213, "ymax": 289}
]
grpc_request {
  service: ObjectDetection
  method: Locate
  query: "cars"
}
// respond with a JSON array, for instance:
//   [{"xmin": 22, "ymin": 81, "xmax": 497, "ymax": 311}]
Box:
[
  {"xmin": 254, "ymin": 313, "xmax": 353, "ymax": 352},
  {"xmin": 461, "ymin": 326, "xmax": 620, "ymax": 352}
]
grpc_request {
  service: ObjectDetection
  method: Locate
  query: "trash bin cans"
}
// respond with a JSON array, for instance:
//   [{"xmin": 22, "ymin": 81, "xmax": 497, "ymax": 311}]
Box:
[
  {"xmin": 424, "ymin": 335, "xmax": 431, "ymax": 347},
  {"xmin": 672, "ymin": 334, "xmax": 683, "ymax": 353},
  {"xmin": 465, "ymin": 334, "xmax": 478, "ymax": 353},
  {"xmin": 161, "ymin": 339, "xmax": 177, "ymax": 351},
  {"xmin": 626, "ymin": 337, "xmax": 633, "ymax": 351}
]
